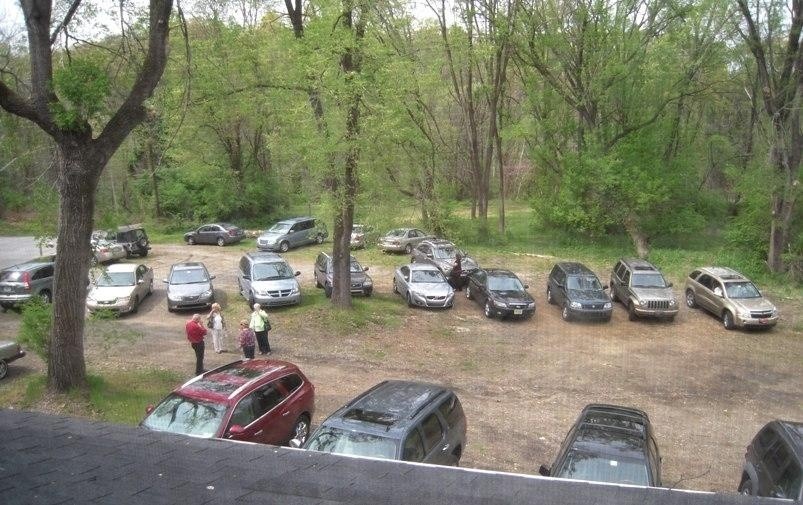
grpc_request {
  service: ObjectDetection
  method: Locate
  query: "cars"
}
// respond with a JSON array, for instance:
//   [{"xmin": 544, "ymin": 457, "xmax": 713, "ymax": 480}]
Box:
[
  {"xmin": 256, "ymin": 217, "xmax": 328, "ymax": 253},
  {"xmin": 377, "ymin": 227, "xmax": 437, "ymax": 255},
  {"xmin": 313, "ymin": 250, "xmax": 377, "ymax": 300},
  {"xmin": 392, "ymin": 263, "xmax": 456, "ymax": 310},
  {"xmin": 183, "ymin": 222, "xmax": 243, "ymax": 247},
  {"xmin": 87, "ymin": 233, "xmax": 127, "ymax": 267},
  {"xmin": 350, "ymin": 224, "xmax": 367, "ymax": 250},
  {"xmin": 163, "ymin": 261, "xmax": 217, "ymax": 312},
  {"xmin": 465, "ymin": 268, "xmax": 536, "ymax": 321},
  {"xmin": 86, "ymin": 263, "xmax": 156, "ymax": 315}
]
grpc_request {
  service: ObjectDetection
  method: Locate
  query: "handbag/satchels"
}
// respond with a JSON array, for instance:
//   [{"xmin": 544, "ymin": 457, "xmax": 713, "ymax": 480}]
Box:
[{"xmin": 264, "ymin": 321, "xmax": 271, "ymax": 332}]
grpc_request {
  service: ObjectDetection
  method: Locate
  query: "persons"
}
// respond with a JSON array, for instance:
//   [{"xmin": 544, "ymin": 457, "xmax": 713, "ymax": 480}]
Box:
[
  {"xmin": 249, "ymin": 302, "xmax": 272, "ymax": 356},
  {"xmin": 206, "ymin": 302, "xmax": 228, "ymax": 354},
  {"xmin": 452, "ymin": 254, "xmax": 462, "ymax": 291},
  {"xmin": 185, "ymin": 313, "xmax": 210, "ymax": 376},
  {"xmin": 238, "ymin": 319, "xmax": 256, "ymax": 359}
]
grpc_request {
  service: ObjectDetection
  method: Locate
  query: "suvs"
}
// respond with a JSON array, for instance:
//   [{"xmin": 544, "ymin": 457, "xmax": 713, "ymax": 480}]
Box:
[
  {"xmin": 103, "ymin": 226, "xmax": 152, "ymax": 259},
  {"xmin": 410, "ymin": 239, "xmax": 478, "ymax": 282},
  {"xmin": 238, "ymin": 252, "xmax": 302, "ymax": 310},
  {"xmin": 287, "ymin": 379, "xmax": 467, "ymax": 473},
  {"xmin": 684, "ymin": 266, "xmax": 778, "ymax": 331},
  {"xmin": 537, "ymin": 402, "xmax": 663, "ymax": 487},
  {"xmin": 547, "ymin": 261, "xmax": 614, "ymax": 323},
  {"xmin": 610, "ymin": 259, "xmax": 680, "ymax": 321},
  {"xmin": 138, "ymin": 356, "xmax": 315, "ymax": 450},
  {"xmin": 736, "ymin": 419, "xmax": 802, "ymax": 503},
  {"xmin": 1, "ymin": 261, "xmax": 91, "ymax": 313}
]
